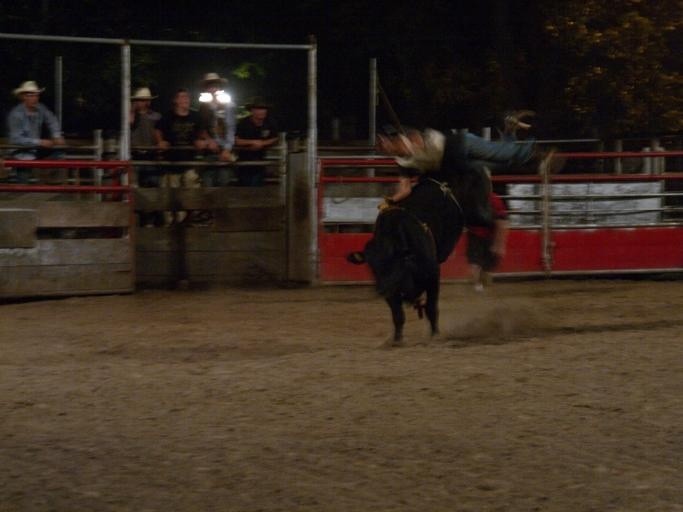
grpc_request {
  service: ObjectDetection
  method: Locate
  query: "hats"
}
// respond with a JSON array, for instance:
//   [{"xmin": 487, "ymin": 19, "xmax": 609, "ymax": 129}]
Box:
[
  {"xmin": 130, "ymin": 88, "xmax": 157, "ymax": 99},
  {"xmin": 200, "ymin": 73, "xmax": 227, "ymax": 89},
  {"xmin": 245, "ymin": 96, "xmax": 270, "ymax": 110},
  {"xmin": 12, "ymin": 80, "xmax": 45, "ymax": 96}
]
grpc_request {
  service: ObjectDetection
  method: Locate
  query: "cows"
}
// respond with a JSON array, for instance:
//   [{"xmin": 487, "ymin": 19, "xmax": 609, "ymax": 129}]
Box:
[{"xmin": 344, "ymin": 108, "xmax": 513, "ymax": 350}]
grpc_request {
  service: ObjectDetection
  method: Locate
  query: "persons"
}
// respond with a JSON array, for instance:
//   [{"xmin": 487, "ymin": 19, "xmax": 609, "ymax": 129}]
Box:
[
  {"xmin": 545, "ymin": 148, "xmax": 578, "ymax": 174},
  {"xmin": 128, "ymin": 86, "xmax": 162, "ymax": 148},
  {"xmin": 234, "ymin": 96, "xmax": 272, "ymax": 186},
  {"xmin": 641, "ymin": 137, "xmax": 666, "ymax": 172},
  {"xmin": 448, "ymin": 109, "xmax": 538, "ymax": 284},
  {"xmin": 6, "ymin": 80, "xmax": 65, "ymax": 160},
  {"xmin": 200, "ymin": 73, "xmax": 237, "ymax": 160},
  {"xmin": 159, "ymin": 88, "xmax": 227, "ymax": 224}
]
